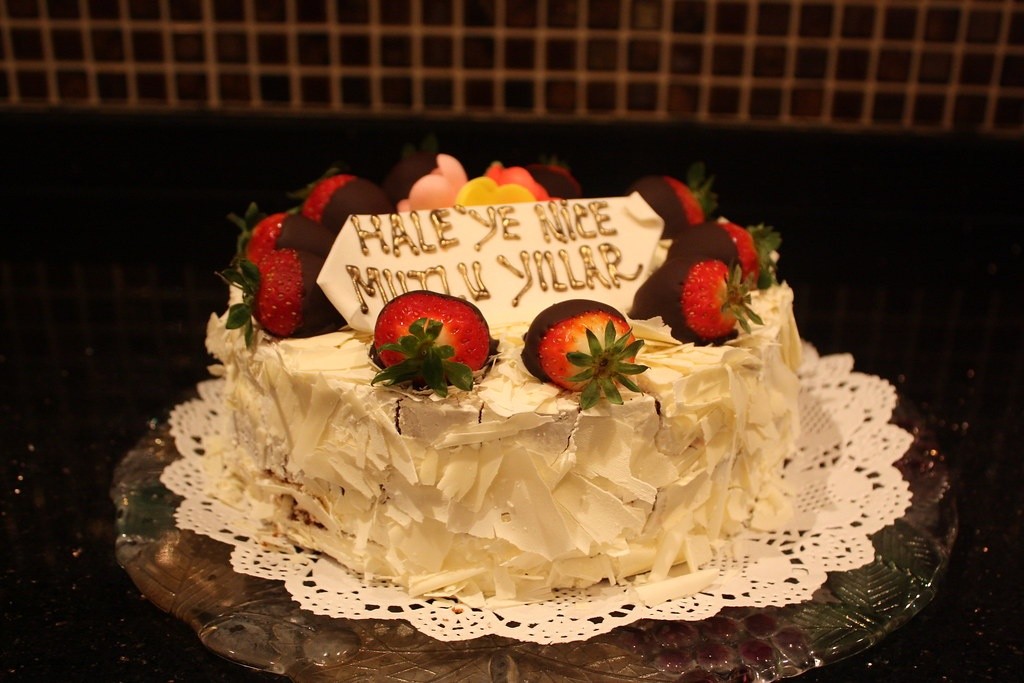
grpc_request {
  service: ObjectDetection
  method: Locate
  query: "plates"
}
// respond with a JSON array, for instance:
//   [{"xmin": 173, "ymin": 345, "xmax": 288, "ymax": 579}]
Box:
[{"xmin": 111, "ymin": 391, "xmax": 959, "ymax": 683}]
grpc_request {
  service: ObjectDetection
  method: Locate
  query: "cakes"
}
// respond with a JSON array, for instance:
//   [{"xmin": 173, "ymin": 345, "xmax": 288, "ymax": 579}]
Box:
[{"xmin": 204, "ymin": 151, "xmax": 803, "ymax": 603}]
